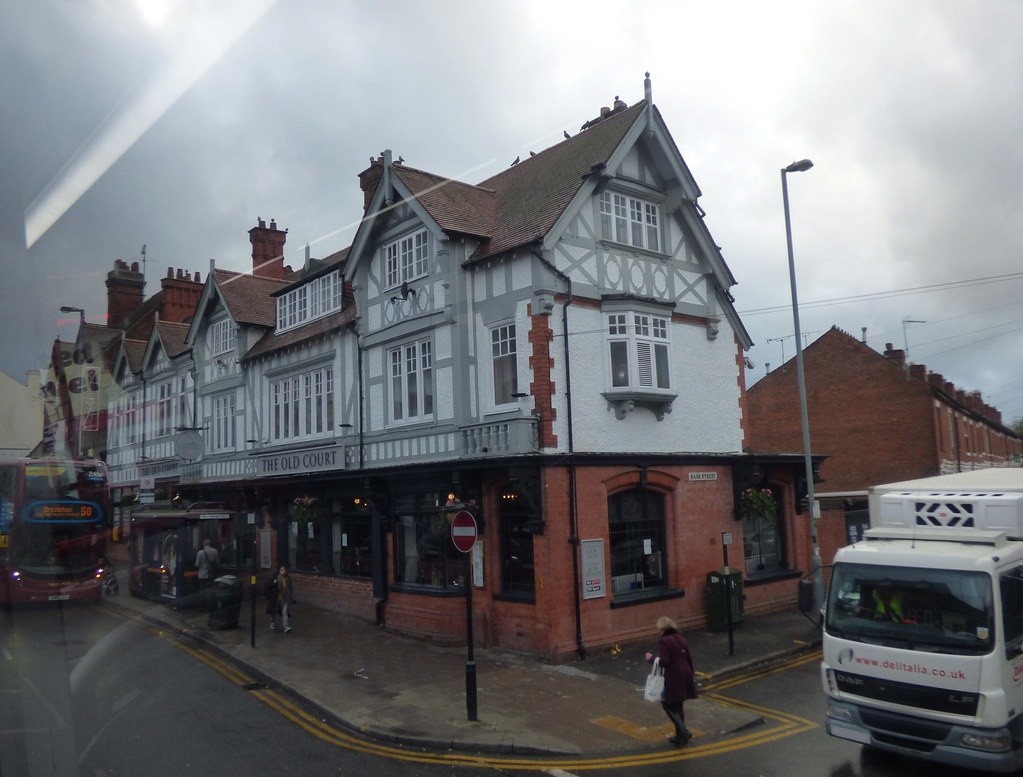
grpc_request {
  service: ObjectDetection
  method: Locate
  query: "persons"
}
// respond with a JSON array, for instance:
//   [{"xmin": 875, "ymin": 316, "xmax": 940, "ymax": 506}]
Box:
[
  {"xmin": 267, "ymin": 564, "xmax": 292, "ymax": 633},
  {"xmin": 195, "ymin": 539, "xmax": 219, "ymax": 611},
  {"xmin": 854, "ymin": 577, "xmax": 912, "ymax": 622},
  {"xmin": 646, "ymin": 616, "xmax": 699, "ymax": 746}
]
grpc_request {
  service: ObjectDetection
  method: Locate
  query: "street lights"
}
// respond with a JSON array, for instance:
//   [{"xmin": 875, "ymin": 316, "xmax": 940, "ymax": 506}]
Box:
[
  {"xmin": 59, "ymin": 304, "xmax": 88, "ymax": 460},
  {"xmin": 780, "ymin": 157, "xmax": 821, "ymax": 641}
]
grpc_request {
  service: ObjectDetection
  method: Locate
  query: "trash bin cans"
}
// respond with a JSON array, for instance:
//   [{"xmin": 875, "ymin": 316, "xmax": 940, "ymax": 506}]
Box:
[{"xmin": 207, "ymin": 574, "xmax": 245, "ymax": 632}]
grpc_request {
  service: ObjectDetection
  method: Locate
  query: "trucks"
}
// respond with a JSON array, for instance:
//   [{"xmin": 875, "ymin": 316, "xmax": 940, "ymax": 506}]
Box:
[{"xmin": 820, "ymin": 467, "xmax": 1023, "ymax": 776}]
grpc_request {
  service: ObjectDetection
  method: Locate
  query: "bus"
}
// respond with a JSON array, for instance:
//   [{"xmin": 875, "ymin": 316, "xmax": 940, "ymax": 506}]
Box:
[{"xmin": 0, "ymin": 454, "xmax": 113, "ymax": 610}]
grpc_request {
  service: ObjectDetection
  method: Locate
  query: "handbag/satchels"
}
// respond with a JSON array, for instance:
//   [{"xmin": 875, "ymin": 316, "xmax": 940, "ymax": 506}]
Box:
[
  {"xmin": 206, "ymin": 560, "xmax": 216, "ymax": 583},
  {"xmin": 643, "ymin": 656, "xmax": 665, "ymax": 703}
]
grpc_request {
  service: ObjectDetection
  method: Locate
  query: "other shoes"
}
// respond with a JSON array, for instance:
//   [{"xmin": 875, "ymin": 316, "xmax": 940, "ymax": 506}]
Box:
[
  {"xmin": 284, "ymin": 626, "xmax": 291, "ymax": 633},
  {"xmin": 270, "ymin": 622, "xmax": 277, "ymax": 629},
  {"xmin": 677, "ymin": 730, "xmax": 692, "ymax": 745},
  {"xmin": 669, "ymin": 736, "xmax": 679, "ymax": 743}
]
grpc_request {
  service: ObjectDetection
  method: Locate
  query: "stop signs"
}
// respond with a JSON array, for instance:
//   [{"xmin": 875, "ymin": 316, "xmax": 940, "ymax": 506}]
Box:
[{"xmin": 450, "ymin": 509, "xmax": 477, "ymax": 553}]
